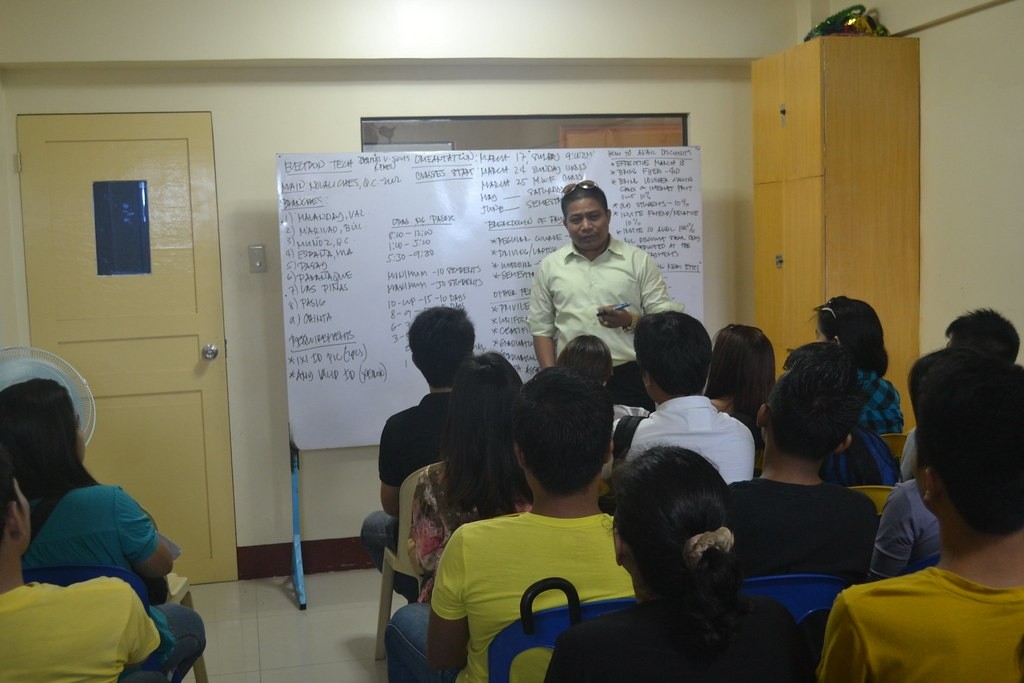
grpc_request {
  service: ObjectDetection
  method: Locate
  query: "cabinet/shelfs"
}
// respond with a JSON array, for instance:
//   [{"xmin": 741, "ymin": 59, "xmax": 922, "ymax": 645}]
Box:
[{"xmin": 751, "ymin": 36, "xmax": 920, "ymax": 434}]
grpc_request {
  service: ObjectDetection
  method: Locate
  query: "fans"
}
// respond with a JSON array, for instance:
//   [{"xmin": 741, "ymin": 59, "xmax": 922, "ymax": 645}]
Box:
[{"xmin": 0, "ymin": 345, "xmax": 98, "ymax": 450}]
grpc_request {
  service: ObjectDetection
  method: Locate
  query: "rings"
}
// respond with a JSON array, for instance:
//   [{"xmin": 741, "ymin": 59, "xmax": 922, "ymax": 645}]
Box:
[{"xmin": 603, "ymin": 321, "xmax": 608, "ymax": 327}]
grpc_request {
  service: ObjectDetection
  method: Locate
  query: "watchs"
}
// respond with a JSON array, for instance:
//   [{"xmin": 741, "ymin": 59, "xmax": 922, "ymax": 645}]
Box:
[{"xmin": 622, "ymin": 311, "xmax": 637, "ymax": 334}]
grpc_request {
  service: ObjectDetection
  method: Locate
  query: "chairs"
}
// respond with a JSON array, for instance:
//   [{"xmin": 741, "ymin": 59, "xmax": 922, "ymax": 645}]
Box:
[
  {"xmin": 373, "ymin": 460, "xmax": 446, "ymax": 660},
  {"xmin": 20, "ymin": 564, "xmax": 209, "ymax": 683},
  {"xmin": 487, "ymin": 434, "xmax": 911, "ymax": 683}
]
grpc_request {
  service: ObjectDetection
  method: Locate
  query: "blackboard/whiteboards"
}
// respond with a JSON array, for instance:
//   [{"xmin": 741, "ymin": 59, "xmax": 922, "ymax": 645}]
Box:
[{"xmin": 274, "ymin": 146, "xmax": 704, "ymax": 449}]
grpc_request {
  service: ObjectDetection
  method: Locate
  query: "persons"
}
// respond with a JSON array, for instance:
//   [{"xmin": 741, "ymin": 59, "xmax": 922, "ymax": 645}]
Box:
[
  {"xmin": 0, "ymin": 447, "xmax": 165, "ymax": 683},
  {"xmin": 384, "ymin": 367, "xmax": 636, "ymax": 682},
  {"xmin": 529, "ymin": 180, "xmax": 671, "ymax": 406},
  {"xmin": 817, "ymin": 347, "xmax": 1024, "ymax": 683},
  {"xmin": 360, "ymin": 295, "xmax": 1021, "ymax": 585},
  {"xmin": 0, "ymin": 377, "xmax": 206, "ymax": 683},
  {"xmin": 542, "ymin": 445, "xmax": 815, "ymax": 683}
]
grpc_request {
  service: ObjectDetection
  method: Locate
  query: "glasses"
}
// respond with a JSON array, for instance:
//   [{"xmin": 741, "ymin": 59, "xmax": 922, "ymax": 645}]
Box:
[
  {"xmin": 561, "ymin": 180, "xmax": 598, "ymax": 197},
  {"xmin": 814, "ymin": 297, "xmax": 837, "ymax": 320}
]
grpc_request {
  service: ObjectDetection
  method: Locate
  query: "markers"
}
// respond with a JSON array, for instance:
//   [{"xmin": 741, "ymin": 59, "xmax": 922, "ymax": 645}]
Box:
[{"xmin": 596, "ymin": 301, "xmax": 630, "ymax": 317}]
